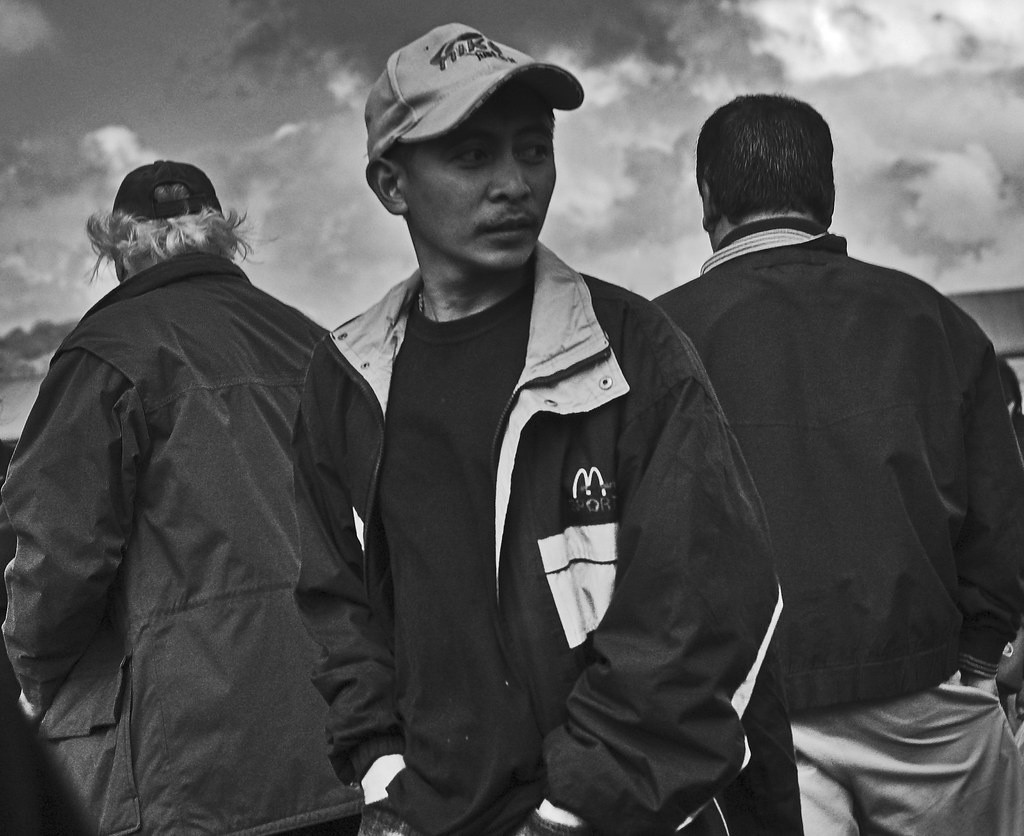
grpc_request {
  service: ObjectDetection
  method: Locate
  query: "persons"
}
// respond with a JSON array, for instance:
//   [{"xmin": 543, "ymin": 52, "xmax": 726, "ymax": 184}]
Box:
[
  {"xmin": 651, "ymin": 92, "xmax": 1024, "ymax": 836},
  {"xmin": 0, "ymin": 158, "xmax": 366, "ymax": 836},
  {"xmin": 292, "ymin": 21, "xmax": 785, "ymax": 836}
]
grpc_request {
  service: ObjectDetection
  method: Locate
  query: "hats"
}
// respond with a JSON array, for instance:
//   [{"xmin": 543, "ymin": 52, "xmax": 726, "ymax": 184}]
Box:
[
  {"xmin": 113, "ymin": 160, "xmax": 222, "ymax": 221},
  {"xmin": 365, "ymin": 22, "xmax": 583, "ymax": 161}
]
